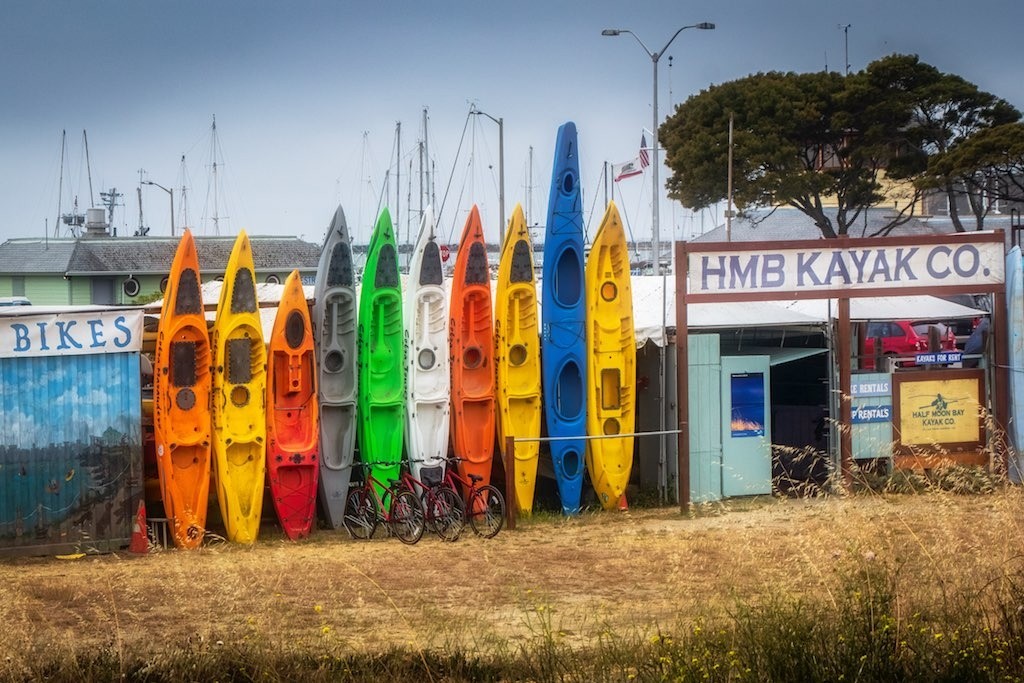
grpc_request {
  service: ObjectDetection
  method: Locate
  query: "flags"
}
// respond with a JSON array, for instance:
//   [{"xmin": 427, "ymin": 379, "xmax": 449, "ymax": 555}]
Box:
[{"xmin": 613, "ymin": 128, "xmax": 650, "ymax": 183}]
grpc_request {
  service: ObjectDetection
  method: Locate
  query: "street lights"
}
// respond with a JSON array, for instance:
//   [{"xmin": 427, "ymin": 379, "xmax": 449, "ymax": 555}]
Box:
[
  {"xmin": 470, "ymin": 111, "xmax": 504, "ymax": 253},
  {"xmin": 603, "ymin": 22, "xmax": 716, "ymax": 276},
  {"xmin": 141, "ymin": 181, "xmax": 174, "ymax": 236}
]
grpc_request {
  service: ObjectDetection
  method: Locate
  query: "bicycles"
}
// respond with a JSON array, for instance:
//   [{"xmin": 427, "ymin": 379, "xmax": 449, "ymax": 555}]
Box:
[
  {"xmin": 343, "ymin": 460, "xmax": 426, "ymax": 545},
  {"xmin": 379, "ymin": 458, "xmax": 466, "ymax": 543},
  {"xmin": 414, "ymin": 456, "xmax": 506, "ymax": 540}
]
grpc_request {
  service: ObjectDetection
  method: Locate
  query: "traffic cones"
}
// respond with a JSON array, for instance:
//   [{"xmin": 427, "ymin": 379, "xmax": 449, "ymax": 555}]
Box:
[{"xmin": 120, "ymin": 498, "xmax": 155, "ymax": 557}]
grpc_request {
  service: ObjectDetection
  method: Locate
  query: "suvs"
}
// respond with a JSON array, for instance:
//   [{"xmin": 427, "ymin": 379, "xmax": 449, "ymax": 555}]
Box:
[{"xmin": 858, "ymin": 318, "xmax": 959, "ymax": 373}]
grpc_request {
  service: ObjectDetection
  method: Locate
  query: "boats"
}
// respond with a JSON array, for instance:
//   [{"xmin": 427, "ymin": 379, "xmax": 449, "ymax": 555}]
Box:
[
  {"xmin": 152, "ymin": 226, "xmax": 214, "ymax": 554},
  {"xmin": 542, "ymin": 121, "xmax": 589, "ymax": 516},
  {"xmin": 449, "ymin": 203, "xmax": 498, "ymax": 522},
  {"xmin": 493, "ymin": 205, "xmax": 542, "ymax": 519},
  {"xmin": 356, "ymin": 207, "xmax": 407, "ymax": 518},
  {"xmin": 211, "ymin": 227, "xmax": 267, "ymax": 549},
  {"xmin": 266, "ymin": 267, "xmax": 321, "ymax": 543},
  {"xmin": 585, "ymin": 196, "xmax": 637, "ymax": 514},
  {"xmin": 401, "ymin": 203, "xmax": 450, "ymax": 524},
  {"xmin": 307, "ymin": 203, "xmax": 360, "ymax": 532}
]
